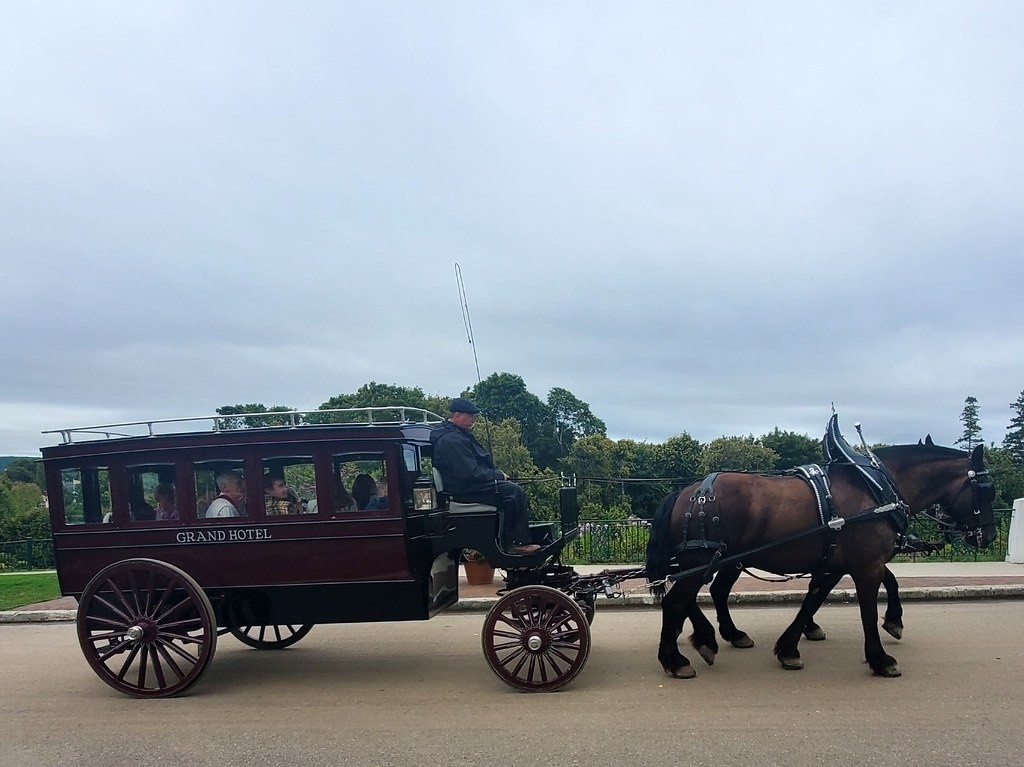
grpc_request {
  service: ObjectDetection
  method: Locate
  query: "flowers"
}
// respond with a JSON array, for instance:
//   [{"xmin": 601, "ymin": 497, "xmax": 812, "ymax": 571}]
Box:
[{"xmin": 462, "ymin": 549, "xmax": 484, "ymax": 565}]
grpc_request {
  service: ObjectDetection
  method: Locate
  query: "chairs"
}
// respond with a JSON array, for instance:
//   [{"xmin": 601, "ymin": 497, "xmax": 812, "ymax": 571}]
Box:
[{"xmin": 432, "ymin": 467, "xmax": 497, "ymax": 513}]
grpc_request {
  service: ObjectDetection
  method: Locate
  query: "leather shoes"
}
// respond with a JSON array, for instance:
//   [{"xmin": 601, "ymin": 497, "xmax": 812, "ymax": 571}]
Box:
[{"xmin": 507, "ymin": 544, "xmax": 541, "ymax": 554}]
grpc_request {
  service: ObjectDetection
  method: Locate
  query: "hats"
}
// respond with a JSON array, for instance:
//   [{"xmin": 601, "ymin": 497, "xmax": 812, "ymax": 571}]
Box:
[{"xmin": 449, "ymin": 399, "xmax": 482, "ymax": 414}]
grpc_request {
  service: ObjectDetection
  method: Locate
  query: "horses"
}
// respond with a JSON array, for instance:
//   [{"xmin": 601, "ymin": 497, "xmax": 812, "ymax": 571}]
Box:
[{"xmin": 643, "ymin": 433, "xmax": 999, "ymax": 679}]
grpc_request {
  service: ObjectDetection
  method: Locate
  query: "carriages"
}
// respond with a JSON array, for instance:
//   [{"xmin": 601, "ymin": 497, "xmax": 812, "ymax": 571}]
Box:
[{"xmin": 41, "ymin": 407, "xmax": 996, "ymax": 700}]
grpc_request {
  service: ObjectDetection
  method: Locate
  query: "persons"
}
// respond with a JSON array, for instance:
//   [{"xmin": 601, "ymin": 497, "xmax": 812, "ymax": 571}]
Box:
[
  {"xmin": 132, "ymin": 461, "xmax": 388, "ymax": 523},
  {"xmin": 429, "ymin": 398, "xmax": 541, "ymax": 554}
]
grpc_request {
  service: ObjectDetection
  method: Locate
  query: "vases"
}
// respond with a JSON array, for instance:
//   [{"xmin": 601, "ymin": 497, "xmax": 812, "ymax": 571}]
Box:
[{"xmin": 465, "ymin": 559, "xmax": 495, "ymax": 583}]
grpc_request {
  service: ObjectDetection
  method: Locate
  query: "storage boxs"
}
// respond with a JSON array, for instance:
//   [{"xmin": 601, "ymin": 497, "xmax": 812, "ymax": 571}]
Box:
[{"xmin": 529, "ymin": 523, "xmax": 556, "ymax": 544}]
